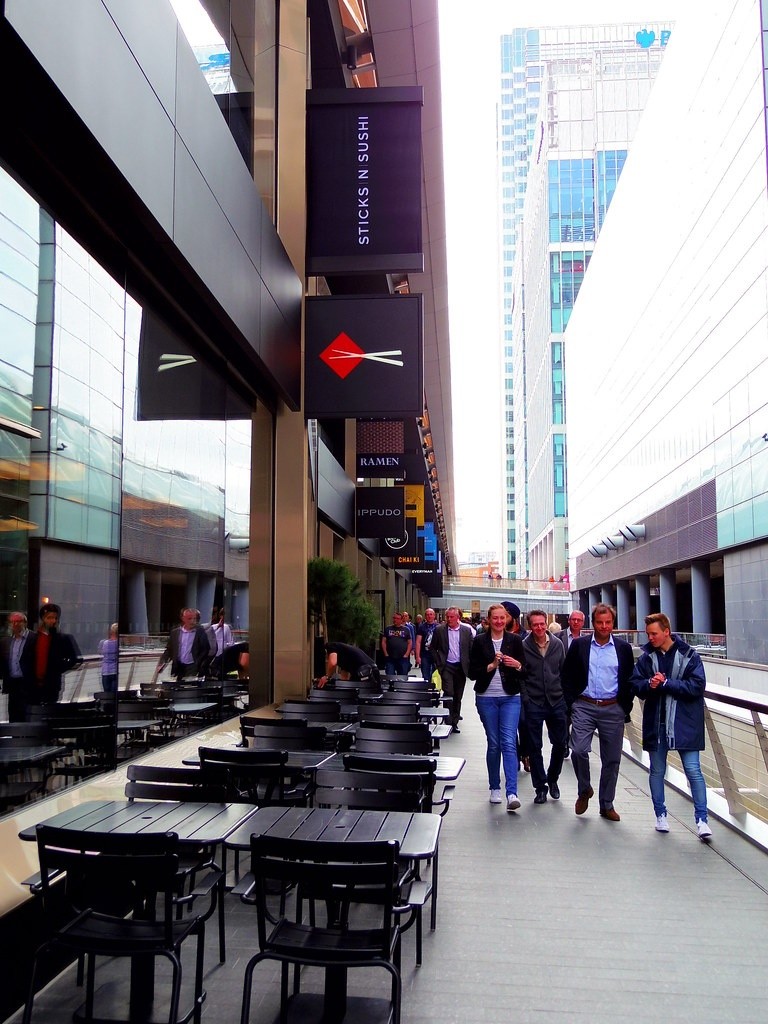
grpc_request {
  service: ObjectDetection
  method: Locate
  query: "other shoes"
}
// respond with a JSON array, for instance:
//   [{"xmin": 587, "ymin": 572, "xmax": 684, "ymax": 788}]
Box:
[
  {"xmin": 600, "ymin": 807, "xmax": 620, "ymax": 820},
  {"xmin": 576, "ymin": 786, "xmax": 593, "ymax": 815},
  {"xmin": 564, "ymin": 739, "xmax": 569, "ymax": 757}
]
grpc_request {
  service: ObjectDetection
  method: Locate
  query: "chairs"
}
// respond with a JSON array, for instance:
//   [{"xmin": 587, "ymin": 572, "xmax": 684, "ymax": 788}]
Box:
[
  {"xmin": 1, "ymin": 678, "xmax": 246, "ymax": 818},
  {"xmin": 26, "ymin": 674, "xmax": 455, "ymax": 1024}
]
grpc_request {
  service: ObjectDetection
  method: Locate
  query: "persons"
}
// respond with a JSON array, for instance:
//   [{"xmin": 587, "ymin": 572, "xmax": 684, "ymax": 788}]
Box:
[
  {"xmin": 560, "ymin": 603, "xmax": 635, "ymax": 822},
  {"xmin": 317, "ymin": 642, "xmax": 381, "ymax": 688},
  {"xmin": 519, "ymin": 609, "xmax": 568, "ymax": 804},
  {"xmin": 489, "ymin": 572, "xmax": 493, "ymax": 587},
  {"xmin": 382, "ymin": 601, "xmax": 588, "ymax": 772},
  {"xmin": 98, "ymin": 622, "xmax": 118, "ymax": 691},
  {"xmin": 627, "ymin": 614, "xmax": 713, "ymax": 838},
  {"xmin": 157, "ymin": 607, "xmax": 250, "ymax": 683},
  {"xmin": 1, "ymin": 604, "xmax": 77, "ymax": 740},
  {"xmin": 542, "ymin": 575, "xmax": 563, "ymax": 589},
  {"xmin": 496, "ymin": 574, "xmax": 502, "ymax": 587},
  {"xmin": 467, "ymin": 603, "xmax": 529, "ymax": 809}
]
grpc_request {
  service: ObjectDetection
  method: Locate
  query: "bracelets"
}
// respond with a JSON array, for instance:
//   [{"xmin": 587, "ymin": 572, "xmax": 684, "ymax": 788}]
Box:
[{"xmin": 325, "ymin": 674, "xmax": 329, "ymax": 679}]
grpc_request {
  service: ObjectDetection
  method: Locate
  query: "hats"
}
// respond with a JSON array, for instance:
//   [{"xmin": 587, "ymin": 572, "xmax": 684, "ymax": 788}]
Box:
[
  {"xmin": 549, "ymin": 623, "xmax": 561, "ymax": 633},
  {"xmin": 501, "ymin": 601, "xmax": 520, "ymax": 619}
]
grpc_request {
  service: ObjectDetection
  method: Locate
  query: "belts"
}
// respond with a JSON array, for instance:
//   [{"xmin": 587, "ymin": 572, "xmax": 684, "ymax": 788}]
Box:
[{"xmin": 578, "ymin": 695, "xmax": 617, "ymax": 706}]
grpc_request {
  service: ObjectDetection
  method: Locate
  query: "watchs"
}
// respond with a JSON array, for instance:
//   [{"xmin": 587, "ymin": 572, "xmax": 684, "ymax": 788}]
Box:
[{"xmin": 516, "ymin": 666, "xmax": 522, "ymax": 672}]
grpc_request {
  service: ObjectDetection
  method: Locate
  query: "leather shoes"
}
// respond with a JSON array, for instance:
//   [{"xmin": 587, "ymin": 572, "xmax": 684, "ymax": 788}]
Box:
[
  {"xmin": 547, "ymin": 775, "xmax": 560, "ymax": 799},
  {"xmin": 522, "ymin": 756, "xmax": 531, "ymax": 771},
  {"xmin": 534, "ymin": 786, "xmax": 548, "ymax": 803},
  {"xmin": 452, "ymin": 725, "xmax": 460, "ymax": 733}
]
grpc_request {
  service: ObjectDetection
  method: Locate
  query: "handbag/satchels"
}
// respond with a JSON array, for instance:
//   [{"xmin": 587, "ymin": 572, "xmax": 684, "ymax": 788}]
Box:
[{"xmin": 431, "ymin": 669, "xmax": 442, "ymax": 692}]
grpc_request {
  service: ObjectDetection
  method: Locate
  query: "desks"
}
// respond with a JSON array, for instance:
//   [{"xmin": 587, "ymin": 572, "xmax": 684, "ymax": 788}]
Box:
[
  {"xmin": 228, "ymin": 805, "xmax": 441, "ymax": 998},
  {"xmin": 340, "ymin": 705, "xmax": 359, "ymax": 720},
  {"xmin": 182, "ymin": 747, "xmax": 337, "ymax": 803},
  {"xmin": 159, "ymin": 702, "xmax": 218, "ymax": 736},
  {"xmin": 20, "ymin": 799, "xmax": 258, "ymax": 1024},
  {"xmin": 117, "ymin": 719, "xmax": 159, "ymax": 740},
  {"xmin": 345, "ymin": 721, "xmax": 453, "ymax": 747},
  {"xmin": 0, "ymin": 747, "xmax": 66, "ymax": 781},
  {"xmin": 316, "ymin": 751, "xmax": 465, "ymax": 799},
  {"xmin": 431, "ymin": 696, "xmax": 453, "ymax": 707},
  {"xmin": 240, "ymin": 722, "xmax": 351, "ymax": 744},
  {"xmin": 417, "ymin": 707, "xmax": 450, "ymax": 725},
  {"xmin": 358, "ymin": 693, "xmax": 382, "ymax": 704}
]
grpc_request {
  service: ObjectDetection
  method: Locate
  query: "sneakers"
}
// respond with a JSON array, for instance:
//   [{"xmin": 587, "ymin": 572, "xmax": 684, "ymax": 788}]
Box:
[
  {"xmin": 697, "ymin": 817, "xmax": 712, "ymax": 838},
  {"xmin": 657, "ymin": 812, "xmax": 669, "ymax": 831},
  {"xmin": 490, "ymin": 789, "xmax": 502, "ymax": 803},
  {"xmin": 507, "ymin": 794, "xmax": 520, "ymax": 810}
]
